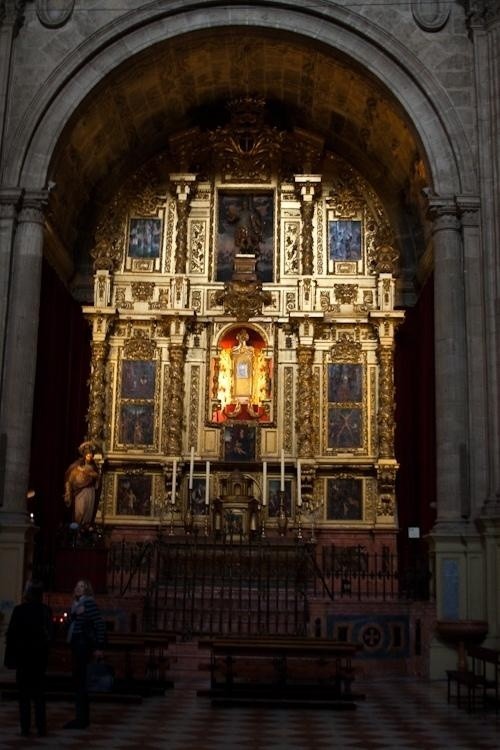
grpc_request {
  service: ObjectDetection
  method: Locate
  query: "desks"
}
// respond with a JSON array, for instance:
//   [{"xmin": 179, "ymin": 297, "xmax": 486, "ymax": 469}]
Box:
[{"xmin": 157, "ymin": 533, "xmax": 319, "ymax": 593}]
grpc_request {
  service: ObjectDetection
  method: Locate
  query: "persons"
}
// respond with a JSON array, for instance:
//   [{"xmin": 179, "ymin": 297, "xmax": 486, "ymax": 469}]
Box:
[
  {"xmin": 4, "ymin": 579, "xmax": 55, "ymax": 738},
  {"xmin": 226, "ymin": 199, "xmax": 262, "ymax": 254},
  {"xmin": 62, "ymin": 579, "xmax": 105, "ymax": 729},
  {"xmin": 60, "ymin": 452, "xmax": 108, "ymax": 526}
]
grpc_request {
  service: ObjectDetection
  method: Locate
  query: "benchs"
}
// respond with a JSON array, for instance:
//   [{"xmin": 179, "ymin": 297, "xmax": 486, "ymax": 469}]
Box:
[
  {"xmin": 85, "ymin": 632, "xmax": 180, "ymax": 704},
  {"xmin": 445, "ymin": 646, "xmax": 499, "ymax": 713},
  {"xmin": 197, "ymin": 634, "xmax": 359, "ymax": 711}
]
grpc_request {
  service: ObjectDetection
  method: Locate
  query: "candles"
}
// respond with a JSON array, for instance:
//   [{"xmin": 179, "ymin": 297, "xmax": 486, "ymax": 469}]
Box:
[
  {"xmin": 296, "ymin": 458, "xmax": 302, "ymax": 506},
  {"xmin": 262, "ymin": 459, "xmax": 267, "ymax": 505},
  {"xmin": 205, "ymin": 460, "xmax": 210, "ymax": 505},
  {"xmin": 280, "ymin": 450, "xmax": 285, "ymax": 491},
  {"xmin": 188, "ymin": 446, "xmax": 194, "ymax": 489},
  {"xmin": 170, "ymin": 460, "xmax": 177, "ymax": 505}
]
